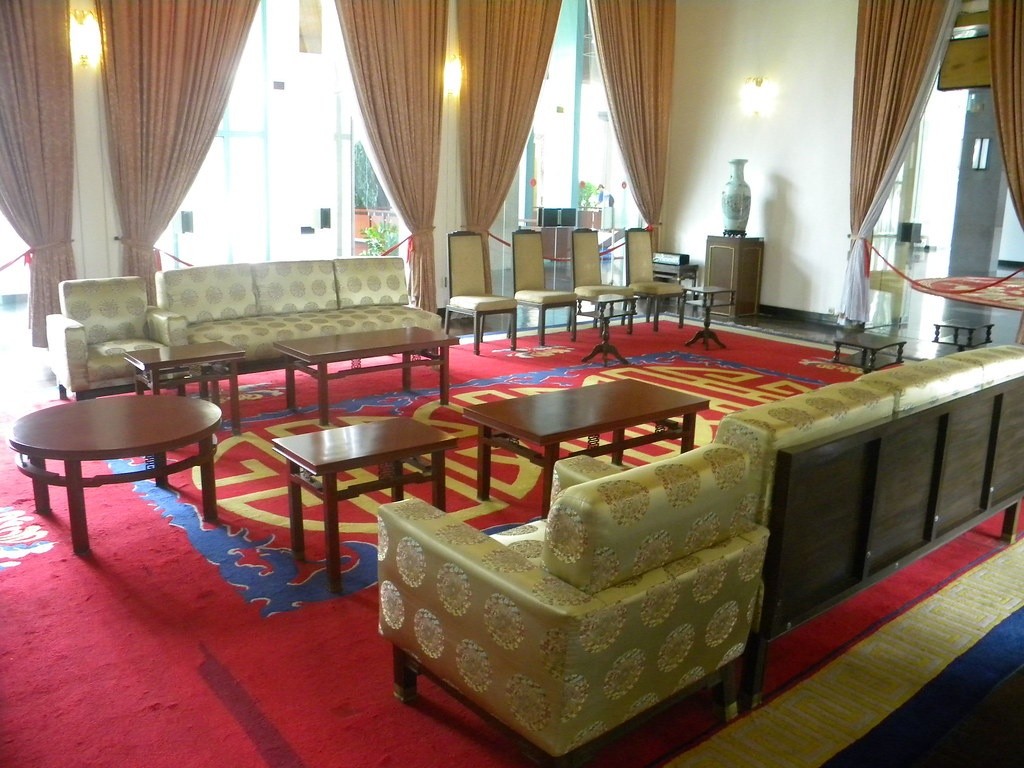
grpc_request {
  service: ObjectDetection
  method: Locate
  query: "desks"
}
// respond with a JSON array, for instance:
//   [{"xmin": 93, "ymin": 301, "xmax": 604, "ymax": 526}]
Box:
[
  {"xmin": 122, "ymin": 340, "xmax": 247, "ymax": 435},
  {"xmin": 463, "ymin": 379, "xmax": 711, "ymax": 520},
  {"xmin": 8, "ymin": 394, "xmax": 223, "ymax": 555},
  {"xmin": 681, "ymin": 286, "xmax": 736, "ymax": 351},
  {"xmin": 571, "ymin": 294, "xmax": 638, "ymax": 369},
  {"xmin": 270, "ymin": 418, "xmax": 458, "ymax": 593},
  {"xmin": 652, "ymin": 262, "xmax": 699, "ymax": 317},
  {"xmin": 273, "ymin": 327, "xmax": 461, "ymax": 426}
]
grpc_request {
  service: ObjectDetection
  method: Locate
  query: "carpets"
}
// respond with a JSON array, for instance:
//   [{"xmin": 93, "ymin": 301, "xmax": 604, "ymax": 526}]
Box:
[{"xmin": 912, "ymin": 268, "xmax": 1024, "ymax": 311}]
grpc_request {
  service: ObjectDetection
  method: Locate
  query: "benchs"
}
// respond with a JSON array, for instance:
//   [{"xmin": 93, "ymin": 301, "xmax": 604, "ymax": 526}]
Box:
[
  {"xmin": 155, "ymin": 256, "xmax": 442, "ymax": 380},
  {"xmin": 46, "ymin": 275, "xmax": 188, "ymax": 402},
  {"xmin": 377, "ymin": 344, "xmax": 1024, "ymax": 768}
]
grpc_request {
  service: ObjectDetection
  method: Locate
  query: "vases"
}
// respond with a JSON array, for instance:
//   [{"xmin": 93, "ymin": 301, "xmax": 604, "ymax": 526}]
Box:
[
  {"xmin": 578, "ymin": 206, "xmax": 602, "ymax": 230},
  {"xmin": 721, "ymin": 158, "xmax": 751, "ymax": 237}
]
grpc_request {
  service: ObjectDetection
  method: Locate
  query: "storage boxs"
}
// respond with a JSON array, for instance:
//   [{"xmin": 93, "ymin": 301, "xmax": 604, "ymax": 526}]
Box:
[{"xmin": 652, "ymin": 252, "xmax": 689, "ymax": 265}]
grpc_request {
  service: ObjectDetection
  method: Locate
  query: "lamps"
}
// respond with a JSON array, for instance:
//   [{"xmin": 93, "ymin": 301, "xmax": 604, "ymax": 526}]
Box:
[
  {"xmin": 740, "ymin": 74, "xmax": 774, "ymax": 118},
  {"xmin": 69, "ymin": 9, "xmax": 102, "ymax": 71},
  {"xmin": 444, "ymin": 53, "xmax": 462, "ymax": 98}
]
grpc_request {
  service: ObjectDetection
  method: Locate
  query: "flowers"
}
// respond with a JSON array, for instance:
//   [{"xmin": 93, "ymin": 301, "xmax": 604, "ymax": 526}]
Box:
[{"xmin": 574, "ymin": 178, "xmax": 601, "ymax": 210}]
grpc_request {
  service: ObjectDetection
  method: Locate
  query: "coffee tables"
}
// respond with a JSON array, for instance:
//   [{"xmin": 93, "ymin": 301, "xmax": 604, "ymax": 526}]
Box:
[
  {"xmin": 931, "ymin": 319, "xmax": 995, "ymax": 352},
  {"xmin": 832, "ymin": 333, "xmax": 907, "ymax": 373}
]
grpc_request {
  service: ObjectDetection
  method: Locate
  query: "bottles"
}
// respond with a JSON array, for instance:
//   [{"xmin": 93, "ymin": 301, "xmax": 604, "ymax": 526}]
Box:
[{"xmin": 723, "ymin": 159, "xmax": 751, "ymax": 231}]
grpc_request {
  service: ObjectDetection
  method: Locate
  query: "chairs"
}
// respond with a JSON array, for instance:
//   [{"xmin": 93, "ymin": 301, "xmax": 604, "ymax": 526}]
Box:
[
  {"xmin": 567, "ymin": 228, "xmax": 638, "ymax": 335},
  {"xmin": 507, "ymin": 229, "xmax": 577, "ymax": 346},
  {"xmin": 621, "ymin": 228, "xmax": 684, "ymax": 332},
  {"xmin": 445, "ymin": 231, "xmax": 517, "ymax": 356}
]
